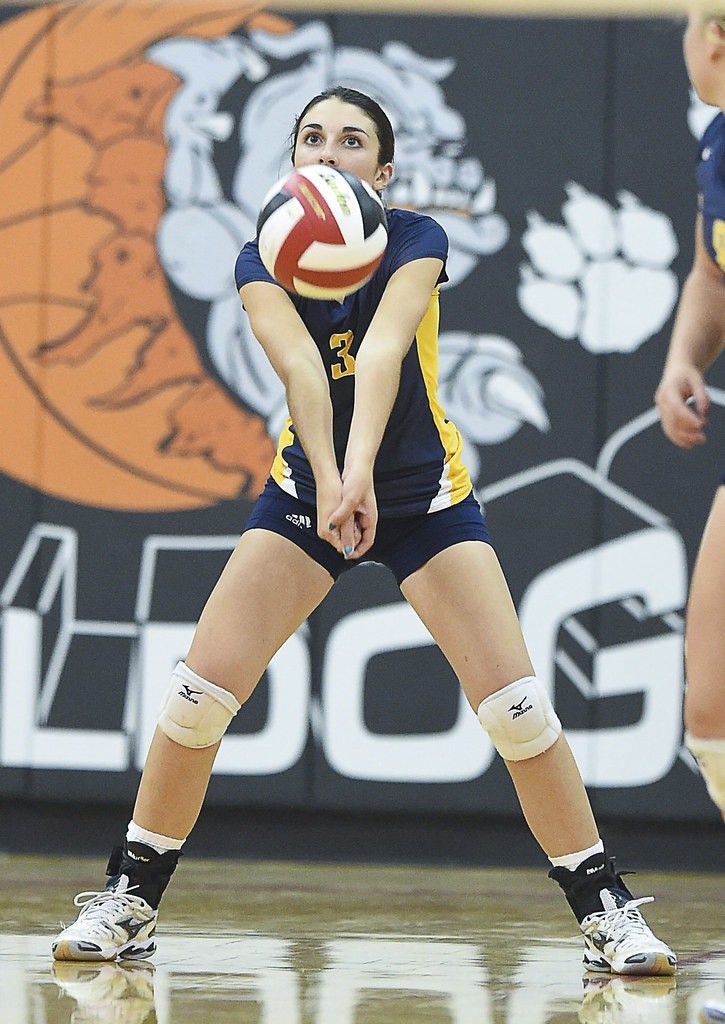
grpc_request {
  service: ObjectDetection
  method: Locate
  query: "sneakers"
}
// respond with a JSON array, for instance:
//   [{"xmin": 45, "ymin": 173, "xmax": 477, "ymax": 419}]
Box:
[
  {"xmin": 50, "ymin": 876, "xmax": 159, "ymax": 964},
  {"xmin": 580, "ymin": 974, "xmax": 679, "ymax": 1024},
  {"xmin": 581, "ymin": 888, "xmax": 679, "ymax": 976},
  {"xmin": 50, "ymin": 959, "xmax": 158, "ymax": 1024}
]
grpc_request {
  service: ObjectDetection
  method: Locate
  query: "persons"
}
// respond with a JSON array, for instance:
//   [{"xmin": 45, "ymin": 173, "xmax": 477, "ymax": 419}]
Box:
[
  {"xmin": 653, "ymin": 1, "xmax": 725, "ymax": 817},
  {"xmin": 51, "ymin": 87, "xmax": 679, "ymax": 975}
]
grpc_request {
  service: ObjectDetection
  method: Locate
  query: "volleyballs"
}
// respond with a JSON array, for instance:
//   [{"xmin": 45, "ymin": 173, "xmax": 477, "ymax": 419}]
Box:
[{"xmin": 256, "ymin": 164, "xmax": 388, "ymax": 300}]
